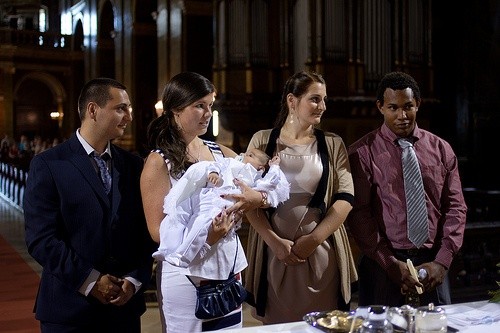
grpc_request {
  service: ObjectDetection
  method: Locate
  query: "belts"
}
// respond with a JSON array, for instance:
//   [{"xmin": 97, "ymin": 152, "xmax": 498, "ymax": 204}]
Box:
[{"xmin": 390, "ymin": 248, "xmax": 437, "ymax": 257}]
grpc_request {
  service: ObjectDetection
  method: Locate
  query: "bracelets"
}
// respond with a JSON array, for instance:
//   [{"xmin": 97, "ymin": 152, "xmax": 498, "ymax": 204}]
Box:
[{"xmin": 260, "ymin": 191, "xmax": 267, "ymax": 209}]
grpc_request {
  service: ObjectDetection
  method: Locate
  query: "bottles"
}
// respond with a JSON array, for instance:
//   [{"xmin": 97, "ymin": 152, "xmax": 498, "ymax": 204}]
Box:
[
  {"xmin": 414, "ymin": 304, "xmax": 447, "ymax": 333},
  {"xmin": 360, "ymin": 305, "xmax": 394, "ymax": 333}
]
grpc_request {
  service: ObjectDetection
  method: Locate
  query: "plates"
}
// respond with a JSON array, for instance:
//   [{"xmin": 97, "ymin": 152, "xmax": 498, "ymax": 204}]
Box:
[{"xmin": 302, "ymin": 311, "xmax": 355, "ymax": 333}]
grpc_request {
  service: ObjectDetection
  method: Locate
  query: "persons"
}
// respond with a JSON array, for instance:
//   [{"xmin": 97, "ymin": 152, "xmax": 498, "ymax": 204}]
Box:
[
  {"xmin": 242, "ymin": 70, "xmax": 359, "ymax": 325},
  {"xmin": 140, "ymin": 71, "xmax": 272, "ymax": 333},
  {"xmin": 0, "ymin": 133, "xmax": 68, "ymax": 164},
  {"xmin": 22, "ymin": 78, "xmax": 159, "ymax": 333},
  {"xmin": 346, "ymin": 72, "xmax": 467, "ymax": 307},
  {"xmin": 152, "ymin": 148, "xmax": 280, "ymax": 268}
]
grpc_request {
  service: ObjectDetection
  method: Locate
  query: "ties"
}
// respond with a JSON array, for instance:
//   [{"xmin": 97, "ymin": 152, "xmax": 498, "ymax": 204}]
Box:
[
  {"xmin": 398, "ymin": 138, "xmax": 430, "ymax": 250},
  {"xmin": 95, "ymin": 156, "xmax": 112, "ymax": 196}
]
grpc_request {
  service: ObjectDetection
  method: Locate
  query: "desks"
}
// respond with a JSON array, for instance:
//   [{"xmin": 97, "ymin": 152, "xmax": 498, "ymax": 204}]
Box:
[{"xmin": 194, "ymin": 297, "xmax": 500, "ymax": 333}]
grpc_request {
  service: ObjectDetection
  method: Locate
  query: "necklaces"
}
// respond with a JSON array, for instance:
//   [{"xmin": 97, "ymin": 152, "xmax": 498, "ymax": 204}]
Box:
[{"xmin": 186, "ymin": 137, "xmax": 200, "ymax": 163}]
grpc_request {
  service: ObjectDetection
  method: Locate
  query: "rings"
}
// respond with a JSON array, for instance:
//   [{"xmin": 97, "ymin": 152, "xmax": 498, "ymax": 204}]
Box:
[
  {"xmin": 284, "ymin": 263, "xmax": 287, "ymax": 265},
  {"xmin": 108, "ymin": 296, "xmax": 113, "ymax": 300},
  {"xmin": 240, "ymin": 210, "xmax": 243, "ymax": 215}
]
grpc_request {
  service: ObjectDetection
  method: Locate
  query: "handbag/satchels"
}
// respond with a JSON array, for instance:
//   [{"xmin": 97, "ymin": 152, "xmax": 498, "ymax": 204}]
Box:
[{"xmin": 196, "ymin": 277, "xmax": 248, "ymax": 320}]
